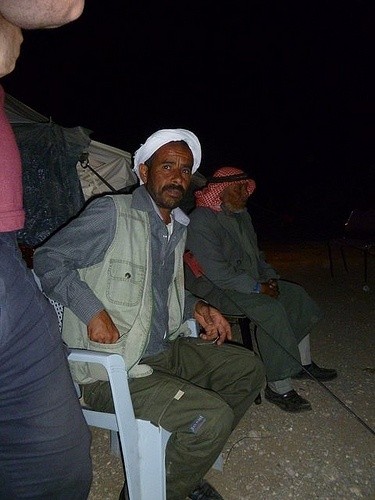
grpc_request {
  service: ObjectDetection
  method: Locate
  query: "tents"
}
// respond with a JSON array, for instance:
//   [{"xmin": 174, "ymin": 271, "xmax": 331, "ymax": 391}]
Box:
[{"xmin": 4, "ymin": 95, "xmax": 139, "ymax": 269}]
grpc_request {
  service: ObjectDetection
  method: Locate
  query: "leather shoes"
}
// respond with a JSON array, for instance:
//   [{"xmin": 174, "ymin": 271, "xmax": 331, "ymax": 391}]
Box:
[
  {"xmin": 264, "ymin": 383, "xmax": 311, "ymax": 413},
  {"xmin": 291, "ymin": 360, "xmax": 337, "ymax": 381},
  {"xmin": 187, "ymin": 479, "xmax": 224, "ymax": 500}
]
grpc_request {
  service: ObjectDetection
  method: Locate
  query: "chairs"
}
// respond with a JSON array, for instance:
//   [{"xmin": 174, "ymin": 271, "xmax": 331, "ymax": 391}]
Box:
[
  {"xmin": 67, "ymin": 319, "xmax": 223, "ymax": 500},
  {"xmin": 218, "ymin": 313, "xmax": 264, "ymax": 404}
]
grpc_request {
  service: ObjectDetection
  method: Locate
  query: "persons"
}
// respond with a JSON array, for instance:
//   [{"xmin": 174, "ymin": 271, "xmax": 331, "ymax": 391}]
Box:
[
  {"xmin": 0, "ymin": 1, "xmax": 95, "ymax": 500},
  {"xmin": 185, "ymin": 166, "xmax": 338, "ymax": 413},
  {"xmin": 32, "ymin": 128, "xmax": 267, "ymax": 500}
]
